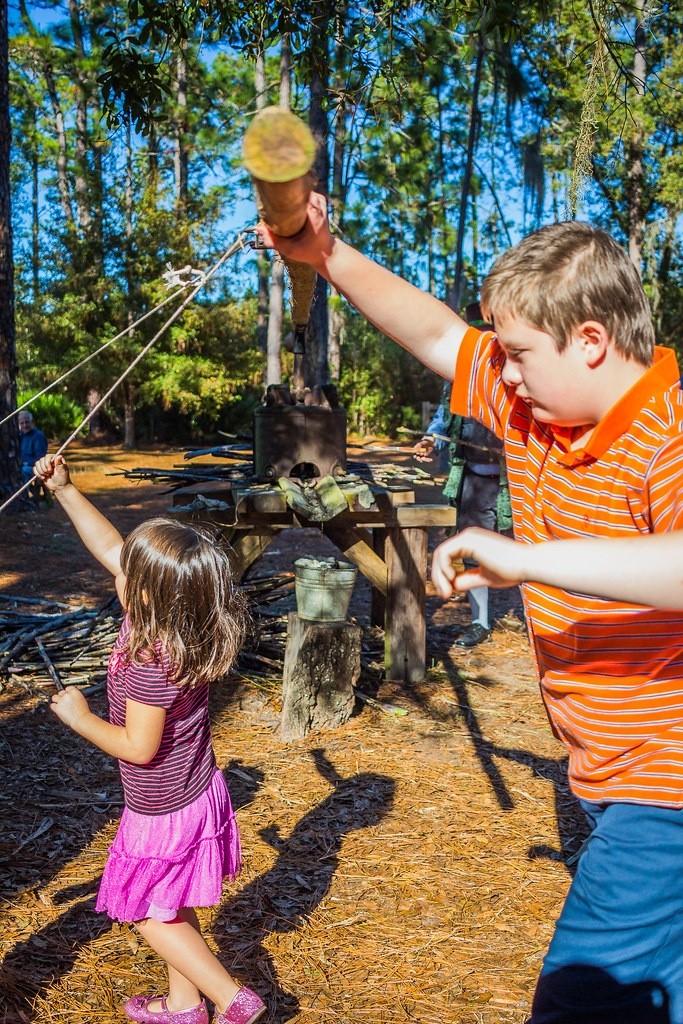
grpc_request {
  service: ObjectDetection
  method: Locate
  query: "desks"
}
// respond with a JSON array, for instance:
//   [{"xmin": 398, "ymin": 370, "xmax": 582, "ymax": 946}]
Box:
[{"xmin": 169, "ymin": 467, "xmax": 457, "ymax": 686}]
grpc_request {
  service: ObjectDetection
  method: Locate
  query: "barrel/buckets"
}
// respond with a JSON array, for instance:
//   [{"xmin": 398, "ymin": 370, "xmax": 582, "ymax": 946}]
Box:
[{"xmin": 295, "ymin": 557, "xmax": 359, "ymax": 622}]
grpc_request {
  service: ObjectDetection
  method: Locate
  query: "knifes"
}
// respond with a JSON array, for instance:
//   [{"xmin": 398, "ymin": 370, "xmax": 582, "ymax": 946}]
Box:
[{"xmin": 362, "ymin": 445, "xmax": 429, "ymax": 453}]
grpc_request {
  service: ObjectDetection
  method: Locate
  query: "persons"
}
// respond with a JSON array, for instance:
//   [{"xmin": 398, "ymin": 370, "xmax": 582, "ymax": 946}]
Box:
[
  {"xmin": 19, "ymin": 411, "xmax": 48, "ymax": 509},
  {"xmin": 32, "ymin": 454, "xmax": 267, "ymax": 1024},
  {"xmin": 412, "ymin": 302, "xmax": 512, "ymax": 648},
  {"xmin": 255, "ymin": 190, "xmax": 682, "ymax": 1024}
]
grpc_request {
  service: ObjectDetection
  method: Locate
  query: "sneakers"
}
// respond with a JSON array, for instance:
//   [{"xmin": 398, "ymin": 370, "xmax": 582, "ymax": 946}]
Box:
[{"xmin": 455, "ymin": 623, "xmax": 492, "ymax": 649}]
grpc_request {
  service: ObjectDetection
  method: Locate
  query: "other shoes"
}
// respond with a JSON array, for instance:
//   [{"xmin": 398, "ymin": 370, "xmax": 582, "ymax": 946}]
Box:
[
  {"xmin": 124, "ymin": 993, "xmax": 208, "ymax": 1024},
  {"xmin": 214, "ymin": 986, "xmax": 266, "ymax": 1024}
]
